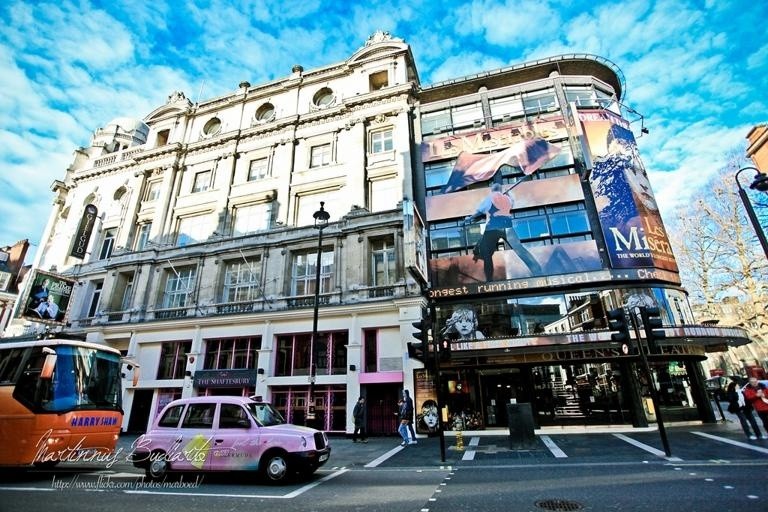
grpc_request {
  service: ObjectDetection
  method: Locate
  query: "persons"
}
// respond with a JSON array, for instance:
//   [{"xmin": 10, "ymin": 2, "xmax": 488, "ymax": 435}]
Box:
[
  {"xmin": 27, "ymin": 278, "xmax": 49, "ymax": 316},
  {"xmin": 446, "ymin": 308, "xmax": 485, "ymax": 339},
  {"xmin": 626, "ymin": 293, "xmax": 654, "ymax": 329},
  {"xmin": 34, "ymin": 295, "xmax": 59, "ymax": 319},
  {"xmin": 745, "ymin": 376, "xmax": 768, "ymax": 433},
  {"xmin": 394, "ymin": 399, "xmax": 410, "ymax": 446},
  {"xmin": 416, "ymin": 400, "xmax": 439, "ymax": 433},
  {"xmin": 466, "ymin": 183, "xmax": 541, "ymax": 281},
  {"xmin": 401, "ymin": 390, "xmax": 418, "ymax": 445},
  {"xmin": 728, "ymin": 383, "xmax": 768, "ymax": 440},
  {"xmin": 353, "ymin": 396, "xmax": 368, "ymax": 443},
  {"xmin": 592, "ymin": 124, "xmax": 658, "ymax": 229}
]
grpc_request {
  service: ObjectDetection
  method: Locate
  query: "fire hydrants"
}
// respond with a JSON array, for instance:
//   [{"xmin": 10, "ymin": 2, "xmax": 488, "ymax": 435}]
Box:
[{"xmin": 456, "ymin": 432, "xmax": 464, "ymax": 450}]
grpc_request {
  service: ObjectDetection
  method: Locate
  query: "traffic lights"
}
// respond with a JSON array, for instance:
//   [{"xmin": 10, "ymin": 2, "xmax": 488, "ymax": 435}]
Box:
[
  {"xmin": 439, "ymin": 340, "xmax": 450, "ymax": 352},
  {"xmin": 606, "ymin": 308, "xmax": 629, "ymax": 343},
  {"xmin": 637, "ymin": 306, "xmax": 666, "ymax": 339},
  {"xmin": 412, "ymin": 318, "xmax": 423, "ymax": 351}
]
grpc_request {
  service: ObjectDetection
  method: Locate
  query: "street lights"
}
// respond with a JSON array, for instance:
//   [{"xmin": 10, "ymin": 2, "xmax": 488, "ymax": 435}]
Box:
[
  {"xmin": 307, "ymin": 202, "xmax": 330, "ymax": 425},
  {"xmin": 735, "ymin": 167, "xmax": 767, "ymax": 259}
]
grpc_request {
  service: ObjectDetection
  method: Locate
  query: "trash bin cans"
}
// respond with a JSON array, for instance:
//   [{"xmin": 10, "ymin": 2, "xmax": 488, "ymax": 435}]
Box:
[{"xmin": 506, "ymin": 403, "xmax": 536, "ymax": 451}]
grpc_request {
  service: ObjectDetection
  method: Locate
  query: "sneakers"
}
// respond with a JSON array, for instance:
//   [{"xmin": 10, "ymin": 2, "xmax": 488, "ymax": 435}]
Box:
[
  {"xmin": 401, "ymin": 440, "xmax": 418, "ymax": 448},
  {"xmin": 749, "ymin": 436, "xmax": 758, "ymax": 440},
  {"xmin": 759, "ymin": 436, "xmax": 768, "ymax": 440}
]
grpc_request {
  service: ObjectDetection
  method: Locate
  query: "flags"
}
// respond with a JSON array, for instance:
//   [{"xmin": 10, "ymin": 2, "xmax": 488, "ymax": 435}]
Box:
[{"xmin": 439, "ymin": 137, "xmax": 561, "ymax": 194}]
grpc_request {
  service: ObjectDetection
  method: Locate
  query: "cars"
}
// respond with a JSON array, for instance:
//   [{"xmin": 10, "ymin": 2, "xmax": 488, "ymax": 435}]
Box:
[
  {"xmin": 132, "ymin": 394, "xmax": 333, "ymax": 482},
  {"xmin": 707, "ymin": 375, "xmax": 744, "ymax": 399},
  {"xmin": 742, "ymin": 379, "xmax": 767, "ymax": 399}
]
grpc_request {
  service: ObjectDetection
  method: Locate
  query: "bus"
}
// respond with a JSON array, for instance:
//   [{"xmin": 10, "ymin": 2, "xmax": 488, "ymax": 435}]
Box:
[{"xmin": 0, "ymin": 339, "xmax": 140, "ymax": 477}]
